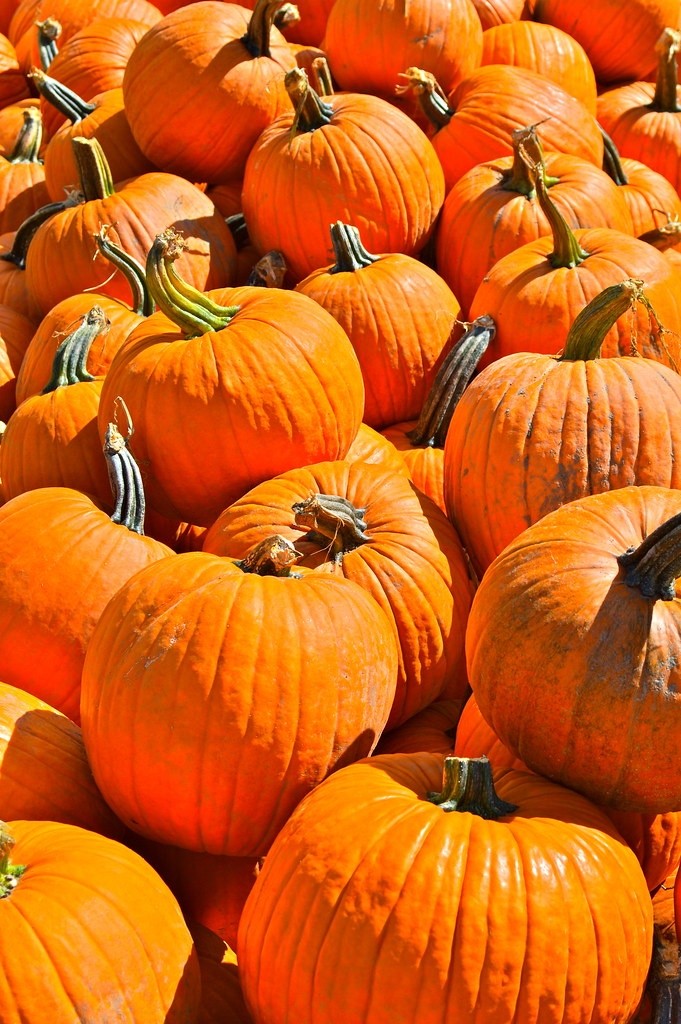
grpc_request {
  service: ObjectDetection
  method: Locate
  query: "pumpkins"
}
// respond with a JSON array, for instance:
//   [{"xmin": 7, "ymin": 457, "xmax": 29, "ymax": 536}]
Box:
[{"xmin": 0, "ymin": 0, "xmax": 681, "ymax": 1024}]
